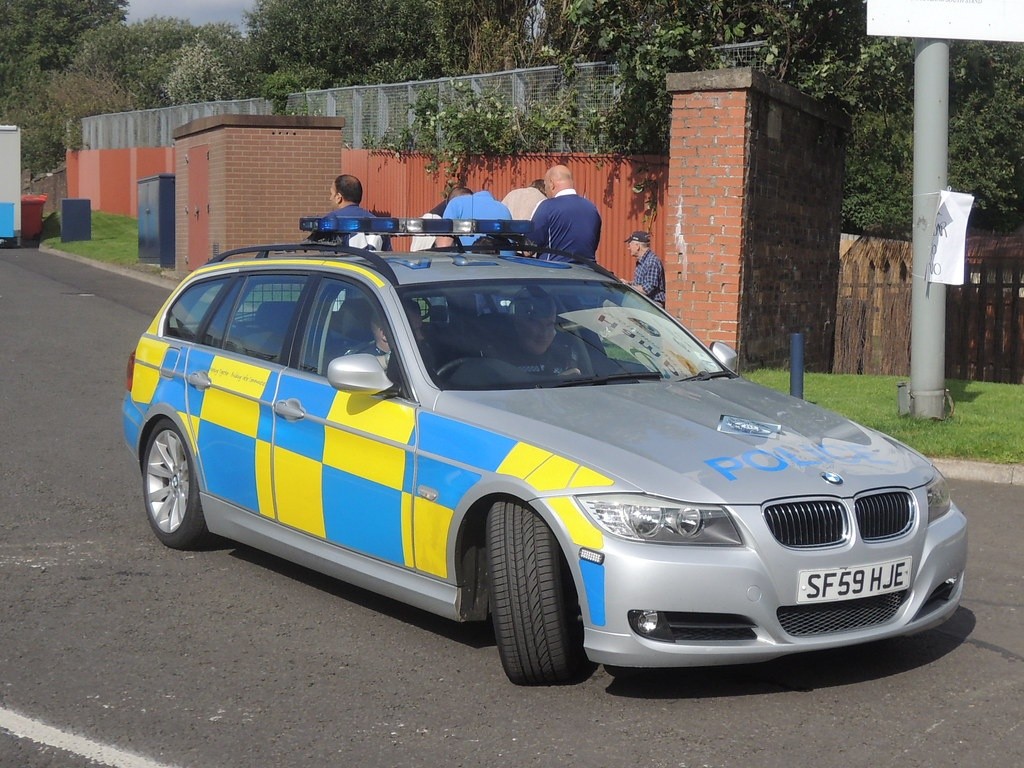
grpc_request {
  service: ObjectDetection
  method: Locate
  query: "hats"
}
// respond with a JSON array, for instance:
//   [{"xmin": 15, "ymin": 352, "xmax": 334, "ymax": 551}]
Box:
[{"xmin": 624, "ymin": 231, "xmax": 650, "ymax": 242}]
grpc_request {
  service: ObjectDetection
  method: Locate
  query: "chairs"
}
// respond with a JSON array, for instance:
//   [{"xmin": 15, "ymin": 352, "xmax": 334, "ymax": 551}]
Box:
[
  {"xmin": 327, "ymin": 300, "xmax": 437, "ymax": 400},
  {"xmin": 471, "ymin": 310, "xmax": 574, "ymax": 383}
]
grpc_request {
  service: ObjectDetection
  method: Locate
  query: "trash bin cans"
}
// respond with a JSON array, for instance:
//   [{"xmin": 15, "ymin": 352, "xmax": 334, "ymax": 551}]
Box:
[{"xmin": 21, "ymin": 193, "xmax": 48, "ymax": 240}]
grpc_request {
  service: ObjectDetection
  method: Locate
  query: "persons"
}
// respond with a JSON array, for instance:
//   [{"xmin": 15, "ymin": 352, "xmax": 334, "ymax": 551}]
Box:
[
  {"xmin": 348, "ymin": 302, "xmax": 443, "ymax": 391},
  {"xmin": 618, "ymin": 231, "xmax": 665, "ymax": 309},
  {"xmin": 300, "ymin": 173, "xmax": 394, "ymax": 253},
  {"xmin": 408, "ymin": 165, "xmax": 603, "ymax": 261},
  {"xmin": 493, "ymin": 287, "xmax": 578, "ymax": 379}
]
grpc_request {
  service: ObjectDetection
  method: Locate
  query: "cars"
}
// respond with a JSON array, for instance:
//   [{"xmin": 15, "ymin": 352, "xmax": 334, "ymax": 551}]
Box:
[{"xmin": 120, "ymin": 214, "xmax": 972, "ymax": 690}]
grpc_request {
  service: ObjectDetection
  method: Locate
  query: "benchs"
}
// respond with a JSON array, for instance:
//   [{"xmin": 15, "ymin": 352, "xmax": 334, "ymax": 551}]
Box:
[{"xmin": 241, "ymin": 295, "xmax": 426, "ymax": 366}]
